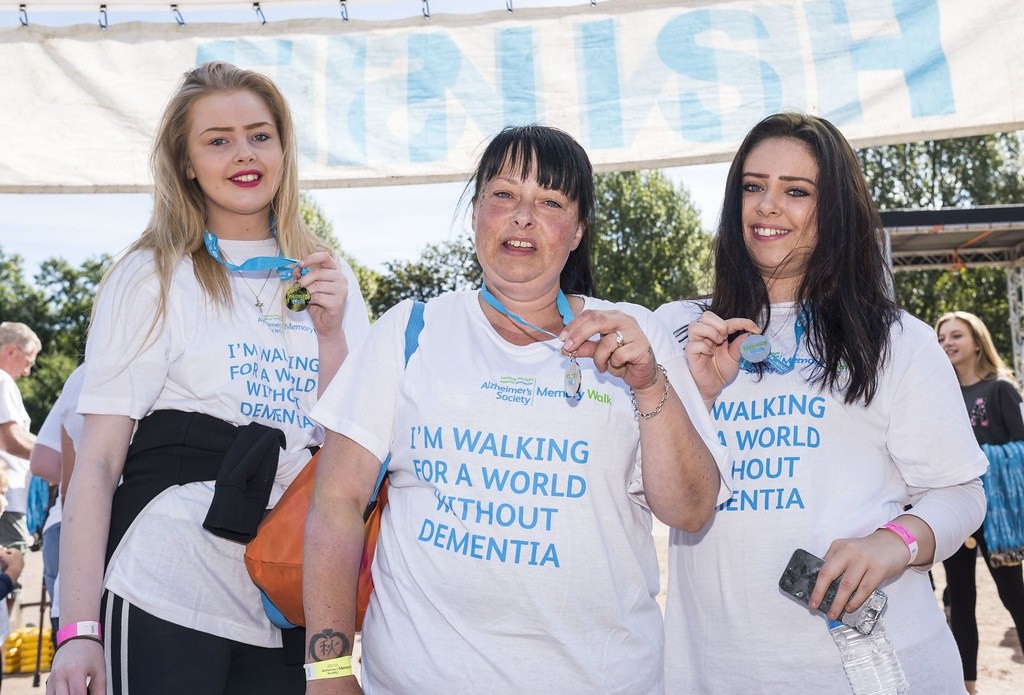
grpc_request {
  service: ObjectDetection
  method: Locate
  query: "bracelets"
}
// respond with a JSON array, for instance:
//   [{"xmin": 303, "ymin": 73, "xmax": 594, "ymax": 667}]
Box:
[
  {"xmin": 54, "ymin": 621, "xmax": 106, "ymax": 651},
  {"xmin": 628, "ymin": 363, "xmax": 670, "ymax": 419},
  {"xmin": 303, "ymin": 655, "xmax": 357, "ymax": 681},
  {"xmin": 874, "ymin": 523, "xmax": 919, "ymax": 565}
]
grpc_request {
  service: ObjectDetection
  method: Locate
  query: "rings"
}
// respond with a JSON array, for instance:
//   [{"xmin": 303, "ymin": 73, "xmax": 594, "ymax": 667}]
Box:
[{"xmin": 615, "ymin": 330, "xmax": 625, "ymax": 347}]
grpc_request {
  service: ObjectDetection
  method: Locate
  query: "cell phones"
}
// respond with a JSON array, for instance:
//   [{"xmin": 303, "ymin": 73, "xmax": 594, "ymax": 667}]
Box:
[{"xmin": 778, "ymin": 548, "xmax": 887, "ymax": 635}]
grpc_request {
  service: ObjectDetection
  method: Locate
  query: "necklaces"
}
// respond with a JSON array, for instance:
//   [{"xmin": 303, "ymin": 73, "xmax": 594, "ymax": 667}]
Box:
[{"xmin": 205, "ymin": 225, "xmax": 281, "ymax": 314}]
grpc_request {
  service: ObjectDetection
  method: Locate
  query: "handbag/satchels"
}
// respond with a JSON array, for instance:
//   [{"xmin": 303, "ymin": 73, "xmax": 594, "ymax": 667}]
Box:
[{"xmin": 243, "ymin": 296, "xmax": 423, "ymax": 632}]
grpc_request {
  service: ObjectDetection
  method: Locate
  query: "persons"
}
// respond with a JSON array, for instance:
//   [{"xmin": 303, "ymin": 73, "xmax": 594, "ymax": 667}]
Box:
[
  {"xmin": 0, "ymin": 319, "xmax": 90, "ymax": 652},
  {"xmin": 931, "ymin": 311, "xmax": 1024, "ymax": 695},
  {"xmin": 46, "ymin": 60, "xmax": 372, "ymax": 695},
  {"xmin": 303, "ymin": 122, "xmax": 734, "ymax": 695},
  {"xmin": 652, "ymin": 113, "xmax": 991, "ymax": 695}
]
